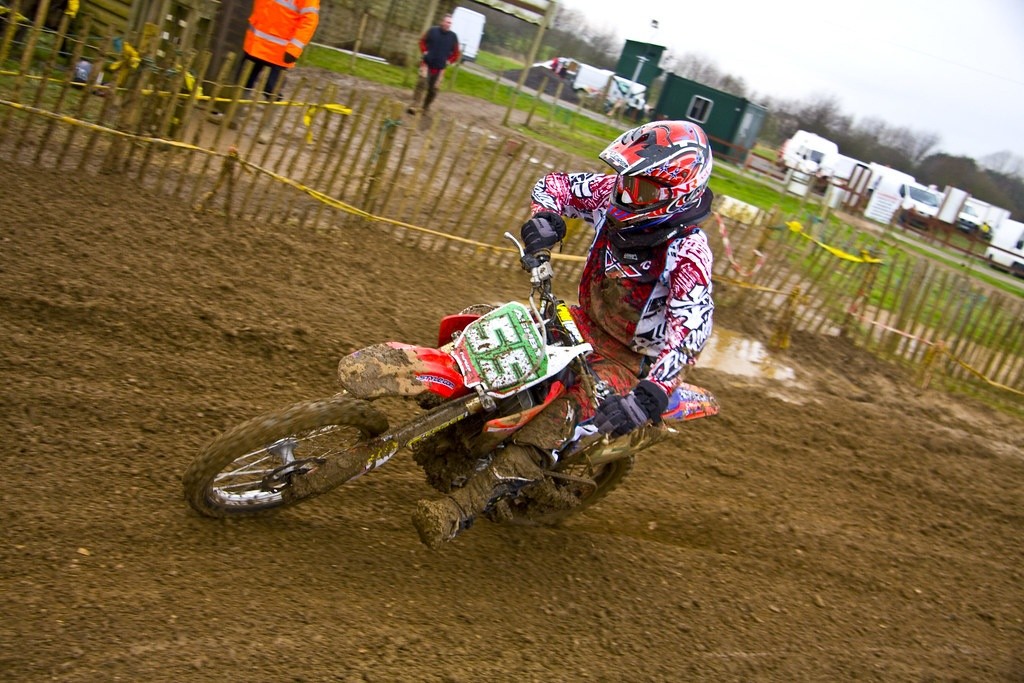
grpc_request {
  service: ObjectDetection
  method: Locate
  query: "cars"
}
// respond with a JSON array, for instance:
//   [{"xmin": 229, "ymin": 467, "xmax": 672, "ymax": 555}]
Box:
[{"xmin": 775, "ymin": 130, "xmax": 1024, "ymax": 276}]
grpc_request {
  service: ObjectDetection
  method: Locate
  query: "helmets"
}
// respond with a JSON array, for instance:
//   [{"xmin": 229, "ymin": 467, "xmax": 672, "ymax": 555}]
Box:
[{"xmin": 598, "ymin": 120, "xmax": 713, "ymax": 231}]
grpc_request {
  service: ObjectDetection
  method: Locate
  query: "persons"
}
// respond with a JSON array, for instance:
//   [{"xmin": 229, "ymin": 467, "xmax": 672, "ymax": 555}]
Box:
[
  {"xmin": 205, "ymin": 0, "xmax": 319, "ymax": 145},
  {"xmin": 411, "ymin": 120, "xmax": 713, "ymax": 551},
  {"xmin": 550, "ymin": 56, "xmax": 567, "ymax": 79},
  {"xmin": 407, "ymin": 13, "xmax": 461, "ymax": 117}
]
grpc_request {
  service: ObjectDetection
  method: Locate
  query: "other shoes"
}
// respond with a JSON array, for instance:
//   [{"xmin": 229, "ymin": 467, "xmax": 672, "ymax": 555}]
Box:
[
  {"xmin": 407, "ymin": 105, "xmax": 417, "ymax": 115},
  {"xmin": 411, "ymin": 497, "xmax": 468, "ymax": 551},
  {"xmin": 208, "ymin": 111, "xmax": 239, "ymax": 130},
  {"xmin": 424, "ymin": 110, "xmax": 432, "ymax": 118},
  {"xmin": 259, "ymin": 124, "xmax": 273, "ymax": 144}
]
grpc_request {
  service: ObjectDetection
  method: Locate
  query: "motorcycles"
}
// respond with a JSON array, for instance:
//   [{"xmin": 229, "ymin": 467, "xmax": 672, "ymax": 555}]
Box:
[{"xmin": 182, "ymin": 231, "xmax": 720, "ymax": 527}]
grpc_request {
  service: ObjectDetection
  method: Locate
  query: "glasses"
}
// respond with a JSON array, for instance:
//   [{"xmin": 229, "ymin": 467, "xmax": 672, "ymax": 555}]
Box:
[{"xmin": 616, "ymin": 174, "xmax": 662, "ymax": 206}]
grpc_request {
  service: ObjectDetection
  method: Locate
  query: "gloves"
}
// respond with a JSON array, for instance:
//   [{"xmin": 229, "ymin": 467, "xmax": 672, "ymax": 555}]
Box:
[
  {"xmin": 594, "ymin": 380, "xmax": 661, "ymax": 438},
  {"xmin": 520, "ymin": 212, "xmax": 567, "ymax": 254}
]
